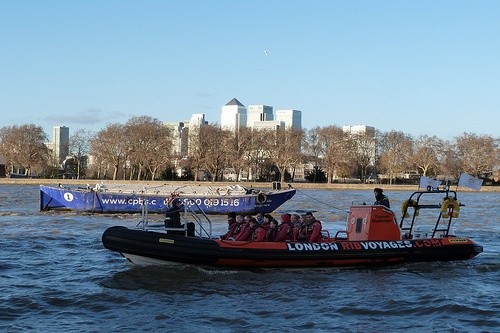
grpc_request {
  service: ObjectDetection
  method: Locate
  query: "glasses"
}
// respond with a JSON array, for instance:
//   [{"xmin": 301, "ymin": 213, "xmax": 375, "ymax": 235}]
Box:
[{"xmin": 307, "ymin": 217, "xmax": 310, "ymax": 218}]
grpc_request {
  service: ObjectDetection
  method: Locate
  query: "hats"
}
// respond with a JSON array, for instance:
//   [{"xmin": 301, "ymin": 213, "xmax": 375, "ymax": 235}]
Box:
[
  {"xmin": 281, "ymin": 214, "xmax": 291, "ymax": 223},
  {"xmin": 172, "ymin": 198, "xmax": 182, "ymax": 205},
  {"xmin": 264, "ymin": 214, "xmax": 273, "ymax": 220},
  {"xmin": 228, "ymin": 212, "xmax": 236, "ymax": 219}
]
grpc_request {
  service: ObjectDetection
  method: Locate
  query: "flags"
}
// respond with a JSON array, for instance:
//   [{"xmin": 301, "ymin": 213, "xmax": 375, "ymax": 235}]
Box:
[
  {"xmin": 419, "ymin": 176, "xmax": 442, "ymax": 189},
  {"xmin": 458, "ymin": 173, "xmax": 483, "ymax": 190}
]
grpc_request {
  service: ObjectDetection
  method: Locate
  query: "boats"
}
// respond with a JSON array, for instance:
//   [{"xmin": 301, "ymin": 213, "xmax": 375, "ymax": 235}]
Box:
[
  {"xmin": 5, "ymin": 172, "xmax": 38, "ymax": 178},
  {"xmin": 102, "ymin": 167, "xmax": 486, "ymax": 274},
  {"xmin": 36, "ymin": 179, "xmax": 298, "ymax": 216}
]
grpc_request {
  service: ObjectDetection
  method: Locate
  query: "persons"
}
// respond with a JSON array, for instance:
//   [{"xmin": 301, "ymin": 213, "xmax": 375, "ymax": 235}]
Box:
[
  {"xmin": 220, "ymin": 212, "xmax": 322, "ymax": 241},
  {"xmin": 372, "ymin": 188, "xmax": 391, "ymax": 209},
  {"xmin": 164, "ymin": 197, "xmax": 185, "ymax": 236}
]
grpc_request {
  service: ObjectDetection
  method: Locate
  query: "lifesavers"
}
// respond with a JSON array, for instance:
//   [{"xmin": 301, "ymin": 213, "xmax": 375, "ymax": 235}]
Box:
[
  {"xmin": 401, "ymin": 199, "xmax": 419, "ymax": 218},
  {"xmin": 441, "ymin": 199, "xmax": 459, "ymax": 218},
  {"xmin": 256, "ymin": 193, "xmax": 267, "ymax": 204}
]
